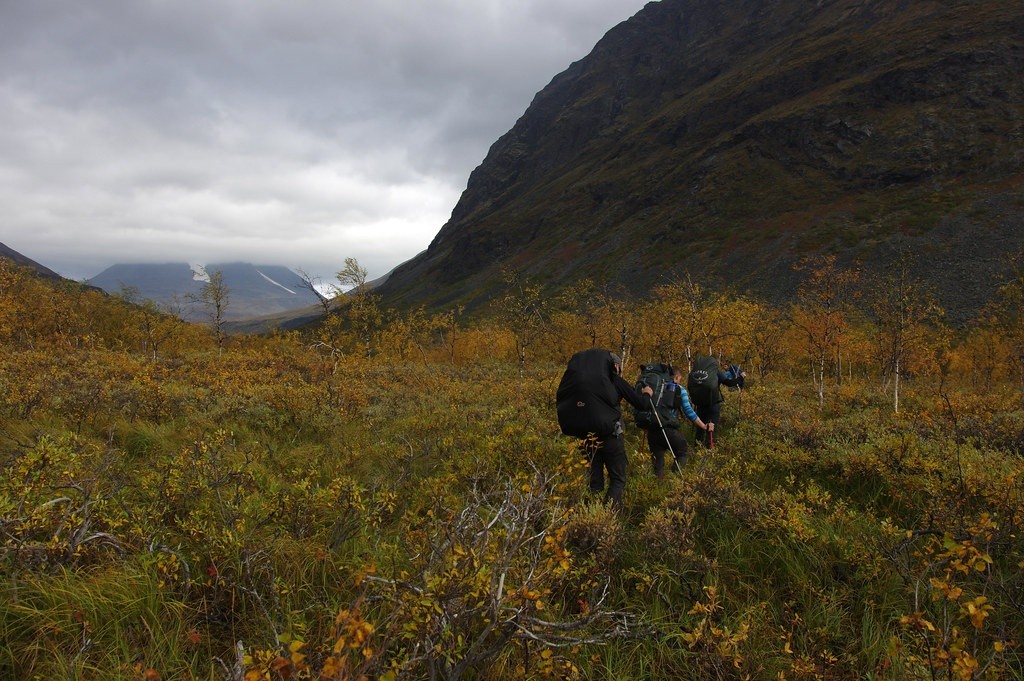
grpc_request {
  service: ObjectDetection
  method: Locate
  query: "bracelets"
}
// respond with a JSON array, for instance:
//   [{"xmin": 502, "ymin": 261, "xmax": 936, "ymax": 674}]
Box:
[{"xmin": 707, "ymin": 423, "xmax": 710, "ymax": 431}]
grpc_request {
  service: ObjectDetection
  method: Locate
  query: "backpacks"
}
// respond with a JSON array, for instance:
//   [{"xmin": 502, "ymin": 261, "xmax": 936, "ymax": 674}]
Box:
[
  {"xmin": 556, "ymin": 347, "xmax": 623, "ymax": 438},
  {"xmin": 729, "ymin": 365, "xmax": 745, "ymax": 386},
  {"xmin": 689, "ymin": 356, "xmax": 722, "ymax": 406},
  {"xmin": 634, "ymin": 362, "xmax": 682, "ymax": 430}
]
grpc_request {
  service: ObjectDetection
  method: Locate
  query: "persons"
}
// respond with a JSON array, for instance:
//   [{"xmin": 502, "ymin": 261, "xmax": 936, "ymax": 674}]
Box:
[
  {"xmin": 724, "ymin": 362, "xmax": 741, "ymax": 391},
  {"xmin": 694, "ymin": 354, "xmax": 747, "ymax": 451},
  {"xmin": 649, "ymin": 364, "xmax": 716, "ymax": 480},
  {"xmin": 583, "ymin": 352, "xmax": 655, "ymax": 512}
]
